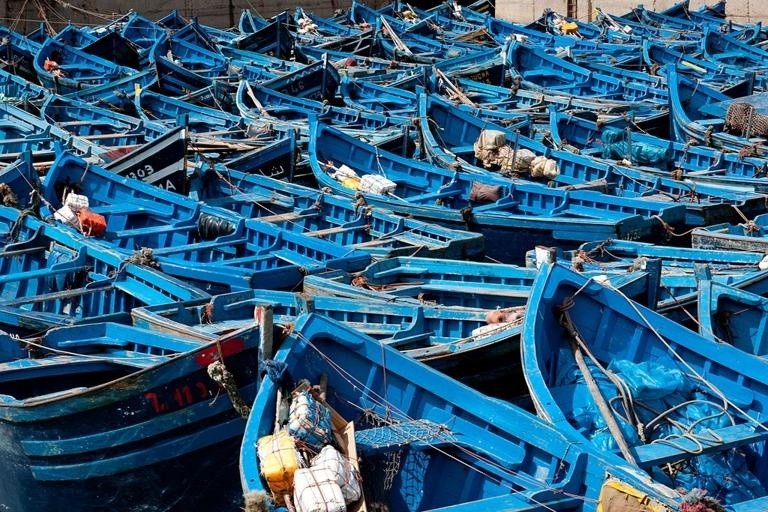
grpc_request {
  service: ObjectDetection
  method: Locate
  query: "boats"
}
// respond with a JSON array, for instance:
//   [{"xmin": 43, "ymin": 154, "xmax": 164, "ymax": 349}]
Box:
[
  {"xmin": 520, "ymin": 251, "xmax": 767, "ymax": 512},
  {"xmin": 238, "ymin": 311, "xmax": 704, "ymax": 512},
  {"xmin": 0, "ymin": 1, "xmax": 768, "ymax": 510},
  {"xmin": 689, "ymin": 264, "xmax": 768, "ymax": 361}
]
